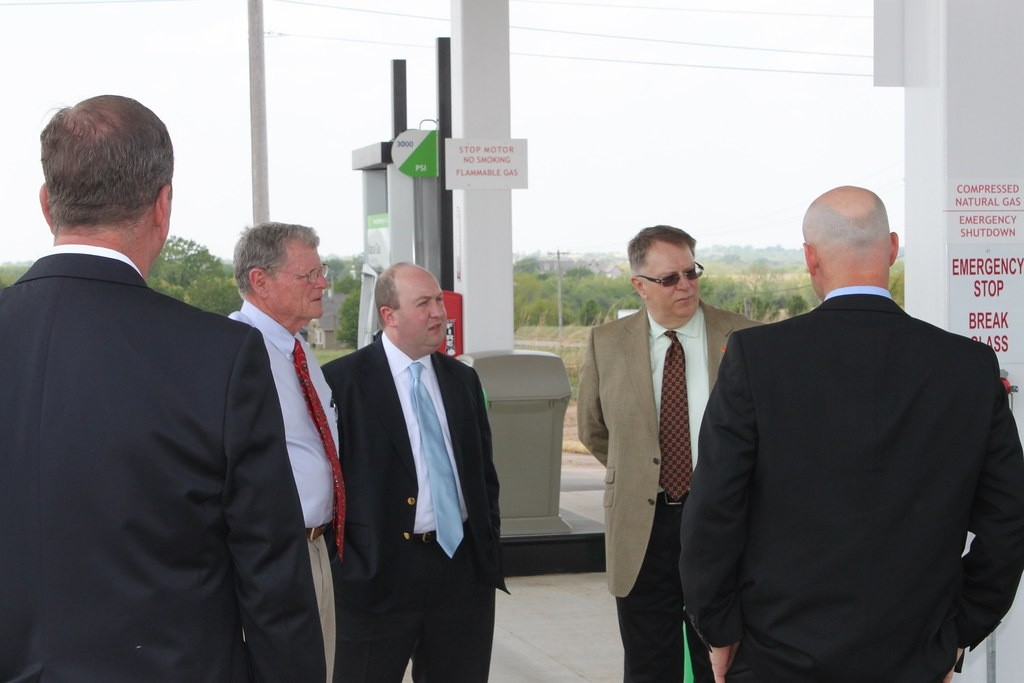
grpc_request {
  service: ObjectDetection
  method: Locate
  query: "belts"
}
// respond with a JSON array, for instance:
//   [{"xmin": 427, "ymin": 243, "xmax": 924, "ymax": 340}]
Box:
[
  {"xmin": 307, "ymin": 523, "xmax": 331, "ymax": 541},
  {"xmin": 412, "ymin": 520, "xmax": 470, "ymax": 545},
  {"xmin": 657, "ymin": 490, "xmax": 692, "ymax": 506}
]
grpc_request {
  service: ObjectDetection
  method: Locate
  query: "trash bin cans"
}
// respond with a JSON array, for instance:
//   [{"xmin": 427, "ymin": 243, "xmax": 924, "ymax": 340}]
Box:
[{"xmin": 455, "ymin": 349, "xmax": 573, "ymax": 519}]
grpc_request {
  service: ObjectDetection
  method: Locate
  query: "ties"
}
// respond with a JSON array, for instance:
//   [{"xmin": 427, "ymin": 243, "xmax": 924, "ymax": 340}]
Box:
[
  {"xmin": 410, "ymin": 359, "xmax": 464, "ymax": 559},
  {"xmin": 293, "ymin": 338, "xmax": 344, "ymax": 564},
  {"xmin": 659, "ymin": 330, "xmax": 693, "ymax": 502}
]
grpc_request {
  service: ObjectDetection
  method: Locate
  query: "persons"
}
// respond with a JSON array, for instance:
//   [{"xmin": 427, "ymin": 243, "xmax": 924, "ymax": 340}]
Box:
[
  {"xmin": 228, "ymin": 224, "xmax": 346, "ymax": 683},
  {"xmin": 576, "ymin": 224, "xmax": 765, "ymax": 683},
  {"xmin": 320, "ymin": 262, "xmax": 511, "ymax": 683},
  {"xmin": 0, "ymin": 94, "xmax": 327, "ymax": 683},
  {"xmin": 678, "ymin": 185, "xmax": 1024, "ymax": 683}
]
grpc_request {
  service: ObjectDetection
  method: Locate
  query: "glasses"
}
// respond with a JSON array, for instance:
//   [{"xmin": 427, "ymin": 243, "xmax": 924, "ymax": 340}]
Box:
[
  {"xmin": 636, "ymin": 260, "xmax": 704, "ymax": 287},
  {"xmin": 255, "ymin": 264, "xmax": 329, "ymax": 283}
]
